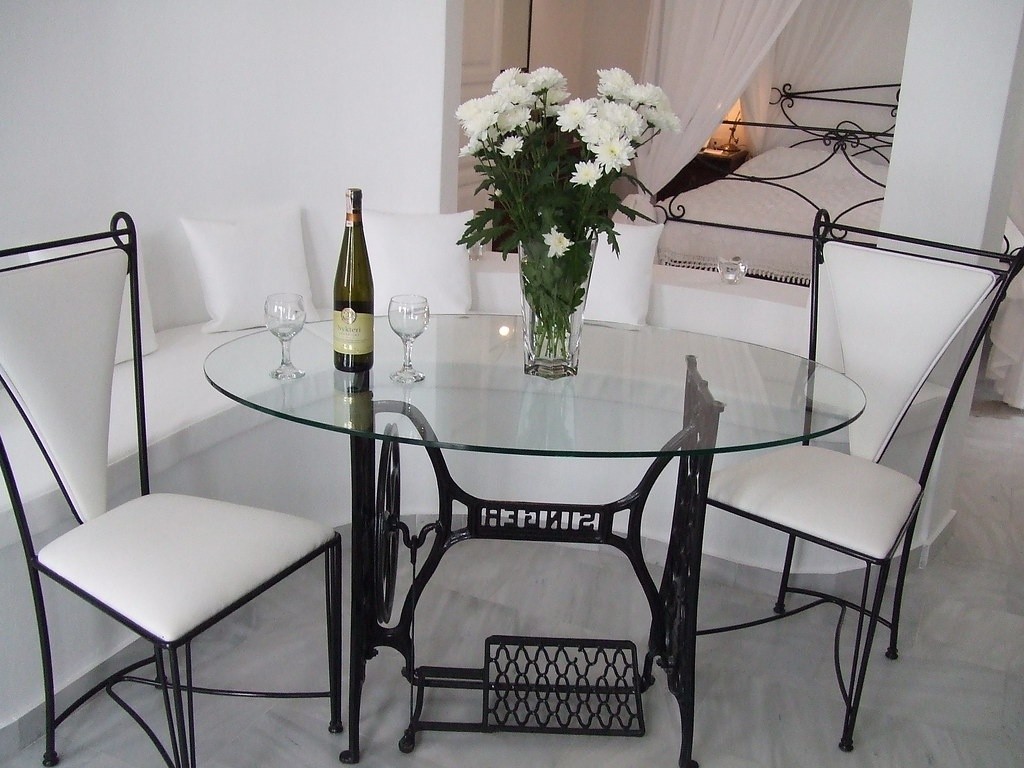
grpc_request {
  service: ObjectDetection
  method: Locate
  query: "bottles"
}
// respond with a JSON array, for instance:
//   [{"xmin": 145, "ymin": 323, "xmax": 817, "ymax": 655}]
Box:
[{"xmin": 333, "ymin": 189, "xmax": 374, "ymax": 373}]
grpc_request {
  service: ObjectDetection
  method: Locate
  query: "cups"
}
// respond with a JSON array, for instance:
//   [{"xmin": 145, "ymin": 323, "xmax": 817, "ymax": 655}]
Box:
[{"xmin": 717, "ymin": 255, "xmax": 748, "ymax": 284}]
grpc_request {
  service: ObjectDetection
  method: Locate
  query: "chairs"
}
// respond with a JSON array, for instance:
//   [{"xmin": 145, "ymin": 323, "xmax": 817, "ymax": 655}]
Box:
[
  {"xmin": 666, "ymin": 204, "xmax": 1024, "ymax": 755},
  {"xmin": 0, "ymin": 209, "xmax": 349, "ymax": 768}
]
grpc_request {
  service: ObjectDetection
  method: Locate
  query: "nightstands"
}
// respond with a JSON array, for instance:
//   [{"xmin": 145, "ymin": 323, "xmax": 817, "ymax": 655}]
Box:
[{"xmin": 686, "ymin": 142, "xmax": 751, "ymax": 188}]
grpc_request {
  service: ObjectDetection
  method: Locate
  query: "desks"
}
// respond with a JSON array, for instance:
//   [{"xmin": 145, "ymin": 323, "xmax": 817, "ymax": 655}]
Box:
[{"xmin": 198, "ymin": 304, "xmax": 868, "ymax": 768}]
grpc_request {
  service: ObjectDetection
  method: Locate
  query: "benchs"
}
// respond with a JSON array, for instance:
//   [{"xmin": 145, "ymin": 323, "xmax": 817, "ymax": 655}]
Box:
[{"xmin": 0, "ymin": 320, "xmax": 970, "ymax": 764}]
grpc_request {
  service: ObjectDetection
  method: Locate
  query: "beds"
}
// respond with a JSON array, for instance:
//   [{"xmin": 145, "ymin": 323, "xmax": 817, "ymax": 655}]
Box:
[{"xmin": 651, "ymin": 83, "xmax": 1024, "ymax": 408}]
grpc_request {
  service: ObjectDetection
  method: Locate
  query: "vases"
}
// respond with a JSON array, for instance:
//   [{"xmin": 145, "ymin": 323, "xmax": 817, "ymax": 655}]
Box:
[{"xmin": 514, "ymin": 230, "xmax": 602, "ymax": 383}]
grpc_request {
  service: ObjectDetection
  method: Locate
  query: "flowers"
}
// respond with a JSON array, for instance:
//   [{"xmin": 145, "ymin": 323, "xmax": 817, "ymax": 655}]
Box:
[{"xmin": 450, "ymin": 63, "xmax": 688, "ymax": 265}]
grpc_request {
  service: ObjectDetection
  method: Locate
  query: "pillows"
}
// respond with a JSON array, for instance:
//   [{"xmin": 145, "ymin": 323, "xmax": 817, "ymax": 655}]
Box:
[
  {"xmin": 26, "ymin": 226, "xmax": 161, "ymax": 361},
  {"xmin": 356, "ymin": 207, "xmax": 476, "ymax": 313},
  {"xmin": 580, "ymin": 219, "xmax": 667, "ymax": 325},
  {"xmin": 174, "ymin": 201, "xmax": 318, "ymax": 336}
]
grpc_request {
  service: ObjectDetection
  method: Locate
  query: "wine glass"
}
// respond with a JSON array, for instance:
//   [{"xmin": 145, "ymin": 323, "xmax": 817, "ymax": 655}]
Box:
[
  {"xmin": 265, "ymin": 294, "xmax": 306, "ymax": 380},
  {"xmin": 388, "ymin": 295, "xmax": 430, "ymax": 383}
]
